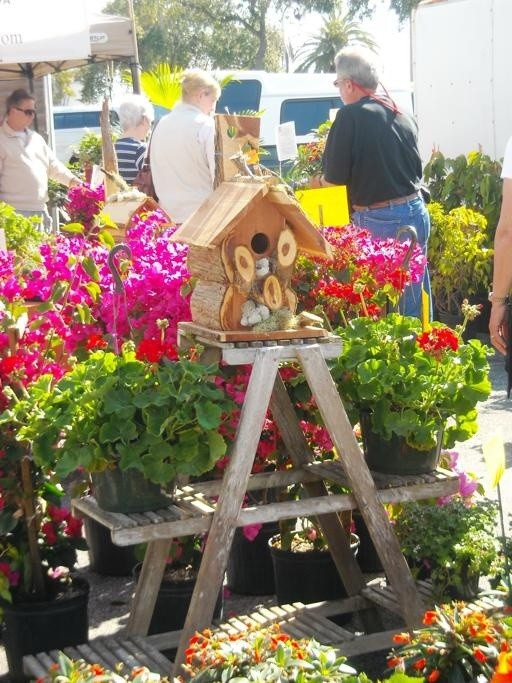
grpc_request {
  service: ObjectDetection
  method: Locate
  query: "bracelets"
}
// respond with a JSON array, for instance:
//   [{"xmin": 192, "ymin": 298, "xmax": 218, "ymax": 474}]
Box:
[
  {"xmin": 488, "ymin": 293, "xmax": 509, "ymax": 304},
  {"xmin": 319, "ymin": 174, "xmax": 324, "ymax": 189}
]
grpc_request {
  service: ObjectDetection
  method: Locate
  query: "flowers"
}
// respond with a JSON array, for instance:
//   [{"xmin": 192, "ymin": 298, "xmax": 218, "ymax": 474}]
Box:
[
  {"xmin": 36, "ymin": 600, "xmax": 512, "ymax": 680},
  {"xmin": 2, "ymin": 505, "xmax": 85, "ymax": 591},
  {"xmin": 418, "ymin": 327, "xmax": 460, "ymax": 365},
  {"xmin": 0, "ymin": 139, "xmax": 428, "ymax": 419}
]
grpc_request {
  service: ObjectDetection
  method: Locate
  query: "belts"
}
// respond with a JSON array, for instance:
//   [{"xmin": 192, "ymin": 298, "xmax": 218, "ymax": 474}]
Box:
[{"xmin": 352, "ymin": 189, "xmax": 423, "ymax": 211}]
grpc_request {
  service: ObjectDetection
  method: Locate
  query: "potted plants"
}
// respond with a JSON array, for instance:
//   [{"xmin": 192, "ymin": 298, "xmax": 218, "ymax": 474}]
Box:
[
  {"xmin": 1, "ymin": 349, "xmax": 228, "ymax": 515},
  {"xmin": 428, "ymin": 139, "xmax": 512, "ymax": 340},
  {"xmin": 323, "ymin": 313, "xmax": 496, "ymax": 471}
]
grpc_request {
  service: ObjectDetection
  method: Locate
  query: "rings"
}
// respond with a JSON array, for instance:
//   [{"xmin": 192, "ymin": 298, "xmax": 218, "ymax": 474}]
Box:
[{"xmin": 491, "ymin": 336, "xmax": 494, "ymax": 339}]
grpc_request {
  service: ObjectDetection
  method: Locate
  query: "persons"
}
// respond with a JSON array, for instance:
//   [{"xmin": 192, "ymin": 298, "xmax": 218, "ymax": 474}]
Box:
[
  {"xmin": 1, "ymin": 88, "xmax": 84, "ymax": 232},
  {"xmin": 488, "ymin": 134, "xmax": 511, "ymax": 400},
  {"xmin": 115, "ymin": 94, "xmax": 154, "ymax": 186},
  {"xmin": 309, "ymin": 45, "xmax": 436, "ymax": 324},
  {"xmin": 133, "ymin": 69, "xmax": 221, "ymax": 224}
]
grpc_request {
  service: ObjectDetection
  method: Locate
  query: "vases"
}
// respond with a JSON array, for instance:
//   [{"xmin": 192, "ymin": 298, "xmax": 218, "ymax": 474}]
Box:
[
  {"xmin": 0, "ymin": 578, "xmax": 91, "ymax": 681},
  {"xmin": 267, "ymin": 533, "xmax": 362, "ymax": 605},
  {"xmin": 132, "ymin": 559, "xmax": 225, "ymax": 637}
]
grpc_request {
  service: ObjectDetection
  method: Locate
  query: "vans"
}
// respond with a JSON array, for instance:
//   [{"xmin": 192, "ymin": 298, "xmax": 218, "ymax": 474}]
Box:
[
  {"xmin": 214, "ymin": 73, "xmax": 342, "ymax": 174},
  {"xmin": 50, "ymin": 107, "xmax": 119, "ymax": 168}
]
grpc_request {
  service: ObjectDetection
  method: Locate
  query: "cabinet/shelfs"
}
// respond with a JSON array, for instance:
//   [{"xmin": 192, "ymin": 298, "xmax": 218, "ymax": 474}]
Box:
[{"xmin": 22, "ymin": 324, "xmax": 506, "ymax": 680}]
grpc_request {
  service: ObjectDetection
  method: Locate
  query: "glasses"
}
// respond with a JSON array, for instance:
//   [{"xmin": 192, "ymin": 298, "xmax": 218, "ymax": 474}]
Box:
[
  {"xmin": 333, "ymin": 79, "xmax": 346, "ymax": 87},
  {"xmin": 13, "ymin": 106, "xmax": 37, "ymax": 116}
]
